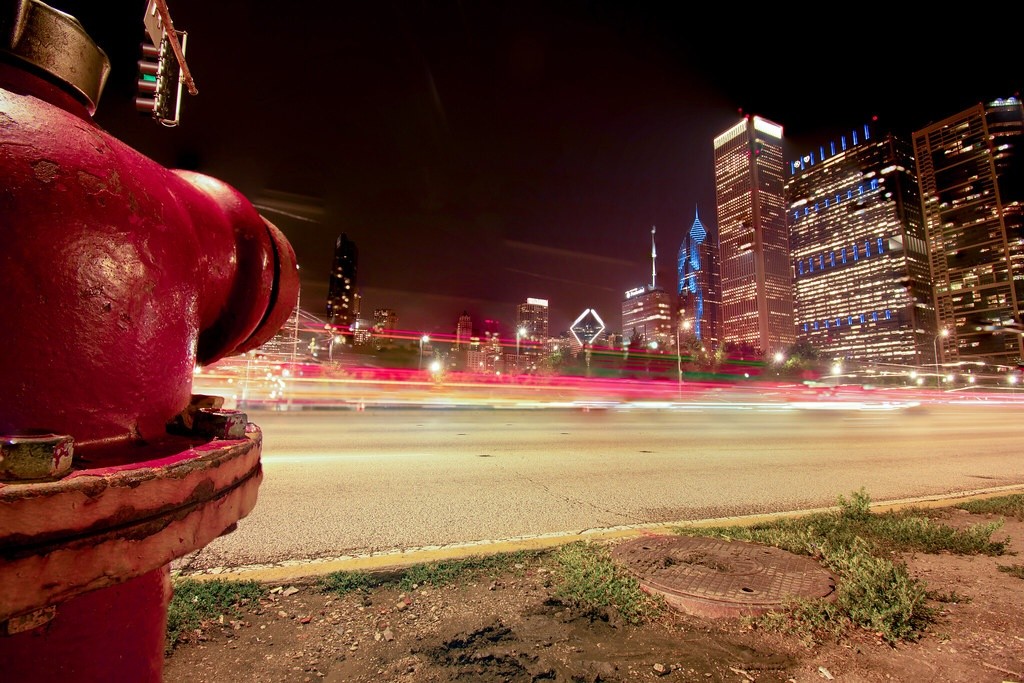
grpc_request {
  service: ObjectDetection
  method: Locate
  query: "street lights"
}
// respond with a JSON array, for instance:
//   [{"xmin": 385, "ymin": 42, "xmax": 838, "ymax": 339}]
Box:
[
  {"xmin": 516, "ymin": 329, "xmax": 527, "ymax": 364},
  {"xmin": 419, "ymin": 335, "xmax": 429, "ymax": 369},
  {"xmin": 934, "ymin": 330, "xmax": 949, "ymax": 389}
]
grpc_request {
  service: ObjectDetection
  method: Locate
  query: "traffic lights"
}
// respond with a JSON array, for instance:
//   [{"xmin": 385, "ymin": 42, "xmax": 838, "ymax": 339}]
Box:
[{"xmin": 132, "ymin": 28, "xmax": 187, "ymax": 126}]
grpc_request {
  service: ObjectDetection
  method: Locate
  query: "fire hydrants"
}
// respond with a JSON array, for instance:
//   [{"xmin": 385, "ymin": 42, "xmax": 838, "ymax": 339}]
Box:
[{"xmin": 0, "ymin": 0, "xmax": 299, "ymax": 683}]
